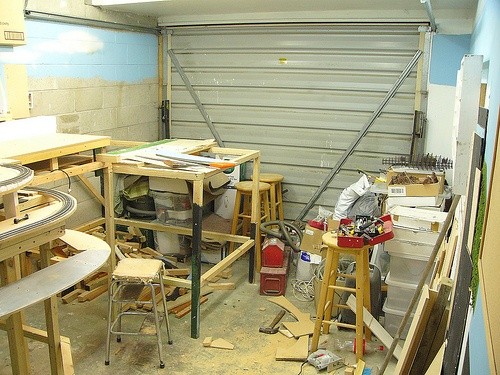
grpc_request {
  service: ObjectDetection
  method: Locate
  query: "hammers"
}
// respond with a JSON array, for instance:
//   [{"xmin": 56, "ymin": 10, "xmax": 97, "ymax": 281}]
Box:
[
  {"xmin": 259, "ymin": 309, "xmax": 286, "ymax": 335},
  {"xmin": 359, "ymin": 215, "xmax": 384, "ymax": 230}
]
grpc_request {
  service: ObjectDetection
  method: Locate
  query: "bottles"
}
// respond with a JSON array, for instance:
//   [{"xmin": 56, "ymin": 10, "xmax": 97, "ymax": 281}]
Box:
[{"xmin": 335, "ymin": 339, "xmax": 384, "ymax": 355}]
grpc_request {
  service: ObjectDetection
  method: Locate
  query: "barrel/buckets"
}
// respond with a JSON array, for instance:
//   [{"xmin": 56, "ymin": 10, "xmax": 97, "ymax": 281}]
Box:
[{"xmin": 341, "ymin": 261, "xmax": 382, "ymax": 327}]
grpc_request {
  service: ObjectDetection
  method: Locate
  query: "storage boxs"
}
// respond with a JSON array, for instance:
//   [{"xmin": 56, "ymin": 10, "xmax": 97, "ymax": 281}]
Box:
[
  {"xmin": 382, "ymin": 170, "xmax": 448, "ymax": 341},
  {"xmin": 300, "ymin": 222, "xmax": 340, "ymax": 263},
  {"xmin": 214, "ymin": 159, "xmax": 246, "ymax": 218},
  {"xmin": 337, "ymin": 215, "xmax": 394, "ymax": 247},
  {"xmin": 148, "ymin": 178, "xmax": 193, "ymax": 262}
]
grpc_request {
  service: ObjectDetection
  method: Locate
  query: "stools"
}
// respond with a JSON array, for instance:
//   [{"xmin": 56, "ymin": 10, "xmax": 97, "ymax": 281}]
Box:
[
  {"xmin": 103, "ymin": 257, "xmax": 172, "ymax": 369},
  {"xmin": 228, "ymin": 173, "xmax": 285, "ymax": 272},
  {"xmin": 311, "ymin": 230, "xmax": 372, "ymax": 362}
]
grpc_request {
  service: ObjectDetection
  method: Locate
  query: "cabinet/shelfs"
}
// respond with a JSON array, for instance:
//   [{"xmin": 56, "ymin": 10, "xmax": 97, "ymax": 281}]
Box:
[{"xmin": 452, "ymin": 54, "xmax": 483, "ymax": 194}]
